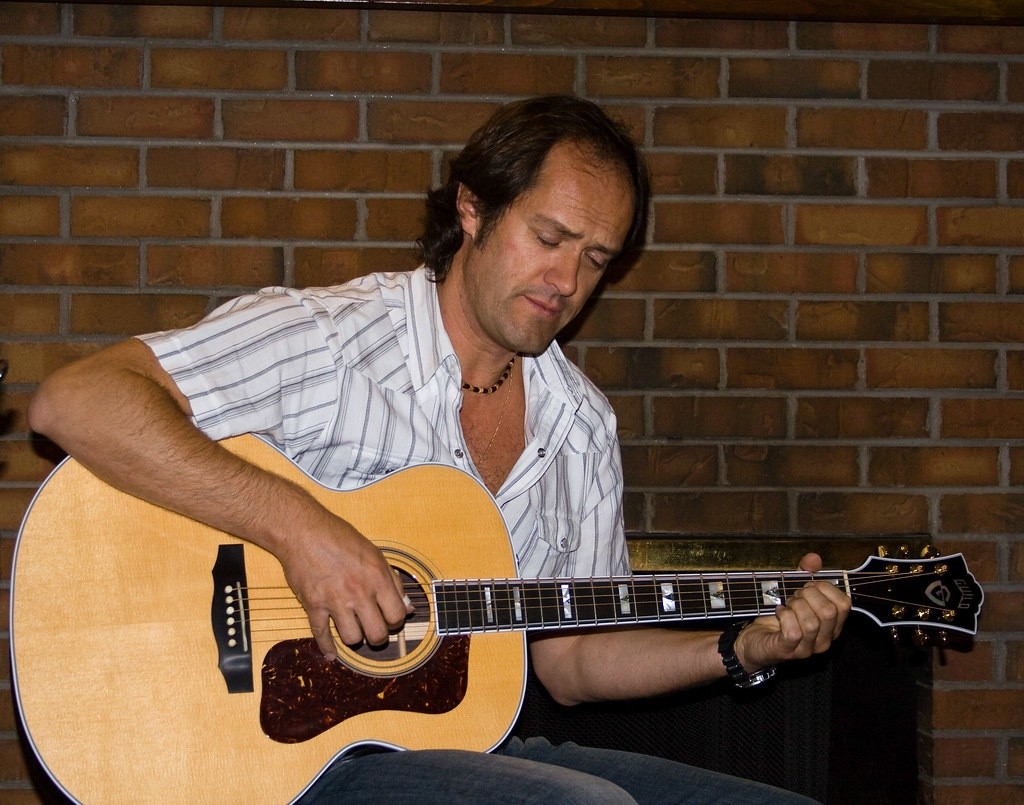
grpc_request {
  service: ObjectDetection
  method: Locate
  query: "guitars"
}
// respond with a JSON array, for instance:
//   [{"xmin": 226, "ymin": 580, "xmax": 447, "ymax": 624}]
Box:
[{"xmin": 6, "ymin": 426, "xmax": 988, "ymax": 805}]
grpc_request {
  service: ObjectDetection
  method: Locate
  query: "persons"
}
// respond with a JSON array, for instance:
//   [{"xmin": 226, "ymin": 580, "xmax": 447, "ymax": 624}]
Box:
[{"xmin": 25, "ymin": 91, "xmax": 825, "ymax": 805}]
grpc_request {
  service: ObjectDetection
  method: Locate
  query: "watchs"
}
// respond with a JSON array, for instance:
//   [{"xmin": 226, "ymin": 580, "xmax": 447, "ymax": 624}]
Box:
[{"xmin": 716, "ymin": 619, "xmax": 778, "ymax": 689}]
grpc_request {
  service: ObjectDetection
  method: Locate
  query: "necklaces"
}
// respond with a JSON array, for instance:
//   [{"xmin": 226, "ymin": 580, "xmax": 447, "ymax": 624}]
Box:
[
  {"xmin": 459, "ymin": 353, "xmax": 517, "ymax": 394},
  {"xmin": 475, "ymin": 369, "xmax": 513, "ymax": 469}
]
